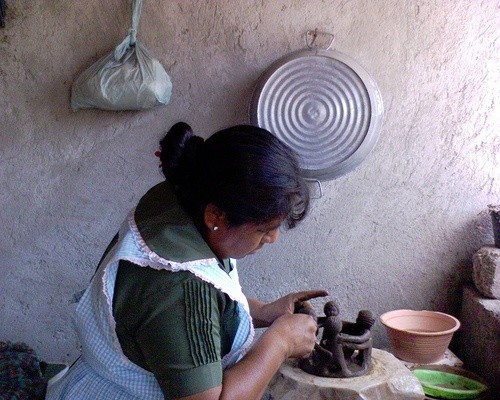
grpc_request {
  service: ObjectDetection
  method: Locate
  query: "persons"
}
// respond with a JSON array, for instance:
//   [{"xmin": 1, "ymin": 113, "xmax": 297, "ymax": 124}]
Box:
[
  {"xmin": 45, "ymin": 122, "xmax": 328, "ymax": 400},
  {"xmin": 303, "ymin": 301, "xmax": 376, "ymax": 378}
]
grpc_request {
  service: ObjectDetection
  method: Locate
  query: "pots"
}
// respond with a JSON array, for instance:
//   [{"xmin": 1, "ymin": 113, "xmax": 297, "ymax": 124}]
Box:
[{"xmin": 249, "ymin": 30, "xmax": 384, "ymax": 199}]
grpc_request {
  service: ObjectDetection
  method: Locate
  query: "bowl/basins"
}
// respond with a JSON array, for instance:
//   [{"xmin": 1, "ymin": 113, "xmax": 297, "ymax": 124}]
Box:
[{"xmin": 380, "ymin": 310, "xmax": 461, "ymax": 363}]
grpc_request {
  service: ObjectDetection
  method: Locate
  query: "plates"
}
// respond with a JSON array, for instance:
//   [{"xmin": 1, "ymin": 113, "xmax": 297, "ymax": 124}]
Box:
[{"xmin": 412, "ymin": 368, "xmax": 487, "ymax": 399}]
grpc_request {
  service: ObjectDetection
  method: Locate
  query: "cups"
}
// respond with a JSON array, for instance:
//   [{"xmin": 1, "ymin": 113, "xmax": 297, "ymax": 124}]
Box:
[{"xmin": 488, "ymin": 204, "xmax": 500, "ymax": 249}]
